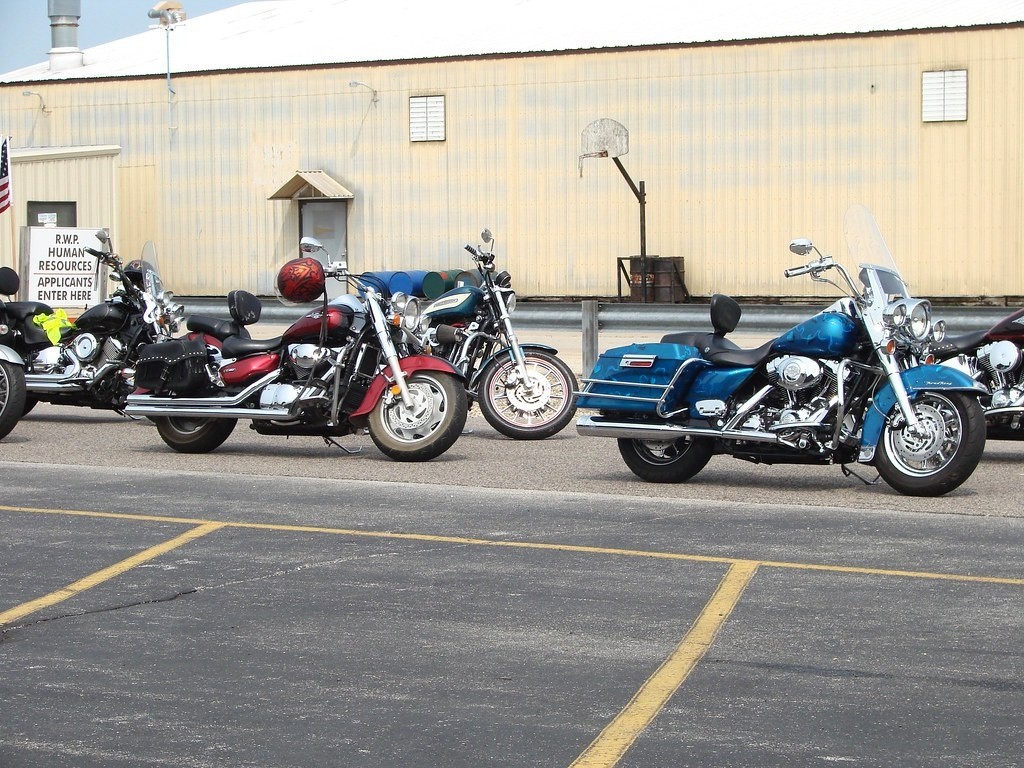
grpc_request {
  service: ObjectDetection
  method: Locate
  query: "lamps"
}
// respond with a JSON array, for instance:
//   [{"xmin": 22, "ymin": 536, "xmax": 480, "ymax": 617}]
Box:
[
  {"xmin": 22, "ymin": 91, "xmax": 51, "ymax": 113},
  {"xmin": 350, "ymin": 82, "xmax": 379, "ymax": 103}
]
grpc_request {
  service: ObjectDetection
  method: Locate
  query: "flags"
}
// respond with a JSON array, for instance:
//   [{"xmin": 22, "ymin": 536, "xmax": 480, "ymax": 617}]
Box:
[{"xmin": 0, "ymin": 138, "xmax": 10, "ymax": 214}]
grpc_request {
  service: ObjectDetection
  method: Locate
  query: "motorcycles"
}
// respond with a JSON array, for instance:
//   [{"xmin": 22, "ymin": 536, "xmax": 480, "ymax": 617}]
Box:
[
  {"xmin": 0, "ymin": 227, "xmax": 185, "ymax": 442},
  {"xmin": 570, "ymin": 203, "xmax": 992, "ymax": 496},
  {"xmin": 124, "ymin": 237, "xmax": 470, "ymax": 464},
  {"xmin": 928, "ymin": 306, "xmax": 1024, "ymax": 442},
  {"xmin": 361, "ymin": 228, "xmax": 580, "ymax": 441}
]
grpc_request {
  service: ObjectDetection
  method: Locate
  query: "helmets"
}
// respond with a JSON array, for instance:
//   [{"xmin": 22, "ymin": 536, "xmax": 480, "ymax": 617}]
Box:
[
  {"xmin": 277, "ymin": 257, "xmax": 325, "ymax": 303},
  {"xmin": 125, "ymin": 260, "xmax": 153, "ymax": 274}
]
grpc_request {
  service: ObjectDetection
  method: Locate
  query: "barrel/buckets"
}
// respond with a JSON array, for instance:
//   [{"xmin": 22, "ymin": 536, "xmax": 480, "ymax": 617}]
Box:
[
  {"xmin": 388, "ymin": 270, "xmax": 428, "ymax": 297},
  {"xmin": 631, "ymin": 255, "xmax": 659, "ymax": 302},
  {"xmin": 653, "ymin": 256, "xmax": 685, "ymax": 302},
  {"xmin": 422, "ymin": 268, "xmax": 465, "ymax": 299},
  {"xmin": 358, "ymin": 269, "xmax": 396, "ymax": 300},
  {"xmin": 453, "ymin": 268, "xmax": 498, "ymax": 289}
]
